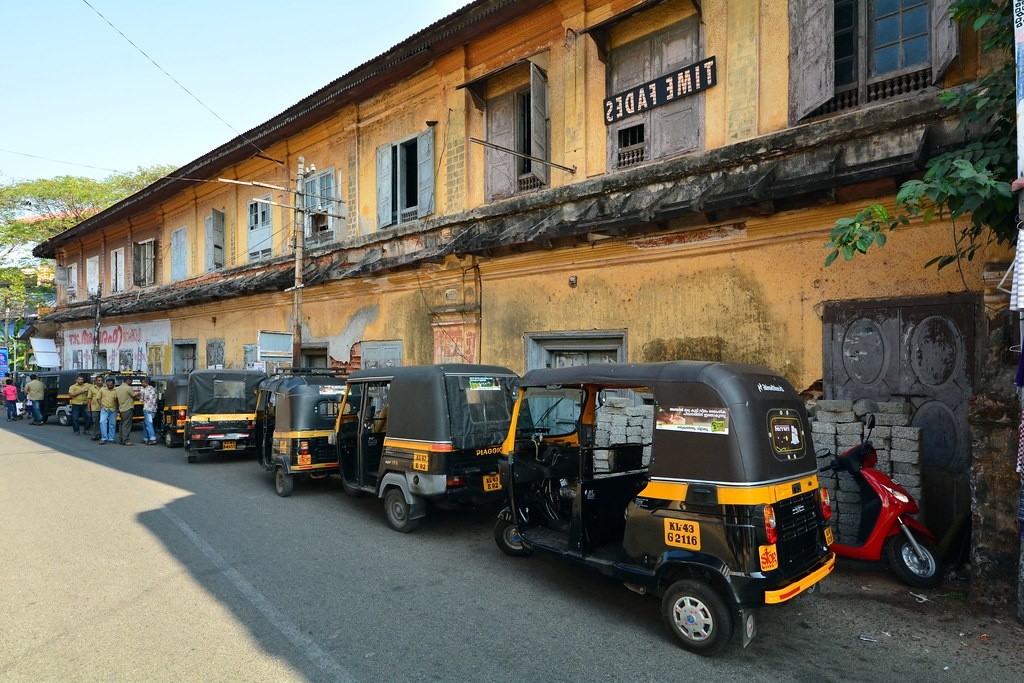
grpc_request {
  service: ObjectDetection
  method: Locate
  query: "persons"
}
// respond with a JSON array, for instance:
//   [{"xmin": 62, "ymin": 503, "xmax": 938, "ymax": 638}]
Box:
[
  {"xmin": 139, "ymin": 379, "xmax": 159, "ymax": 446},
  {"xmin": 68, "ymin": 374, "xmax": 94, "ymax": 436},
  {"xmin": 86, "ymin": 375, "xmax": 107, "ymax": 442},
  {"xmin": 96, "ymin": 378, "xmax": 119, "ymax": 445},
  {"xmin": 24, "ymin": 374, "xmax": 49, "ymax": 426},
  {"xmin": 25, "ymin": 389, "xmax": 34, "ymax": 419},
  {"xmin": 0, "ymin": 372, "xmax": 21, "ymax": 422},
  {"xmin": 116, "ymin": 375, "xmax": 143, "ymax": 446}
]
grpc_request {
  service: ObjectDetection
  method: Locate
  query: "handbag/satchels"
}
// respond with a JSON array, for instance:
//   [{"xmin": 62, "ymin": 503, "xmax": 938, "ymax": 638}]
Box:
[{"xmin": 16, "ymin": 401, "xmax": 25, "ymax": 415}]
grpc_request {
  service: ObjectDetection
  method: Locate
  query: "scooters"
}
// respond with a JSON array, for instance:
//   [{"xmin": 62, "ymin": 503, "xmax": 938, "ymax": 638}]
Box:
[{"xmin": 814, "ymin": 412, "xmax": 944, "ymax": 588}]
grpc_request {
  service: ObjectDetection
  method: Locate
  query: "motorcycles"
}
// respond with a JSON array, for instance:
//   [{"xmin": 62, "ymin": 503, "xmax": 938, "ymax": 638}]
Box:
[
  {"xmin": 158, "ymin": 374, "xmax": 193, "ymax": 448},
  {"xmin": 182, "ymin": 368, "xmax": 270, "ymax": 463},
  {"xmin": 14, "ymin": 370, "xmax": 42, "ymax": 417},
  {"xmin": 253, "ymin": 366, "xmax": 374, "ymax": 497},
  {"xmin": 493, "ymin": 361, "xmax": 835, "ymax": 657},
  {"xmin": 333, "ymin": 363, "xmax": 535, "ymax": 534},
  {"xmin": 88, "ymin": 370, "xmax": 176, "ymax": 439},
  {"xmin": 34, "ymin": 369, "xmax": 112, "ymax": 426}
]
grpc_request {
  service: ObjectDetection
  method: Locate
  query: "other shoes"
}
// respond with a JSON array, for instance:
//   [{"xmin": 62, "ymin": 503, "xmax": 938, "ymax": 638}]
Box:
[
  {"xmin": 148, "ymin": 440, "xmax": 157, "ymax": 444},
  {"xmin": 74, "ymin": 431, "xmax": 80, "ymax": 436},
  {"xmin": 36, "ymin": 420, "xmax": 44, "ymax": 425},
  {"xmin": 109, "ymin": 439, "xmax": 117, "ymax": 443},
  {"xmin": 142, "ymin": 440, "xmax": 149, "ymax": 444},
  {"xmin": 29, "ymin": 421, "xmax": 37, "ymax": 425},
  {"xmin": 83, "ymin": 430, "xmax": 91, "ymax": 434},
  {"xmin": 91, "ymin": 435, "xmax": 100, "ymax": 440},
  {"xmin": 7, "ymin": 417, "xmax": 11, "ymax": 421},
  {"xmin": 99, "ymin": 439, "xmax": 108, "ymax": 444},
  {"xmin": 120, "ymin": 440, "xmax": 134, "ymax": 445}
]
